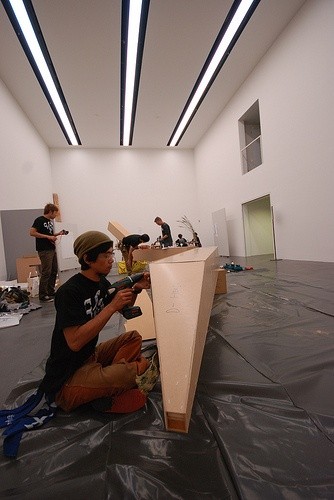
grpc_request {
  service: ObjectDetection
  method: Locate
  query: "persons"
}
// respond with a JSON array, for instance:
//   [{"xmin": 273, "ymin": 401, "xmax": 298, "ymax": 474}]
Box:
[
  {"xmin": 176, "ymin": 234, "xmax": 188, "ymax": 247},
  {"xmin": 119, "ymin": 234, "xmax": 150, "ymax": 277},
  {"xmin": 154, "ymin": 217, "xmax": 173, "ymax": 247},
  {"xmin": 43, "ymin": 231, "xmax": 153, "ymax": 414},
  {"xmin": 191, "ymin": 232, "xmax": 202, "ymax": 247},
  {"xmin": 30, "ymin": 204, "xmax": 67, "ymax": 303}
]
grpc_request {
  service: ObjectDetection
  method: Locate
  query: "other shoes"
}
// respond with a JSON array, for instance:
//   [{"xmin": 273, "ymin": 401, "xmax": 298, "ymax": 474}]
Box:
[{"xmin": 92, "ymin": 389, "xmax": 146, "ymax": 413}]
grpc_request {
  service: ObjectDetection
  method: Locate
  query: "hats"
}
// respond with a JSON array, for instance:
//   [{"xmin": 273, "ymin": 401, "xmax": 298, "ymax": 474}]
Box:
[{"xmin": 73, "ymin": 231, "xmax": 112, "ymax": 260}]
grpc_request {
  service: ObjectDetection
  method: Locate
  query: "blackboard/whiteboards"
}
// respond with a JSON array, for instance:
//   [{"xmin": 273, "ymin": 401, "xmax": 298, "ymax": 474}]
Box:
[
  {"xmin": 212, "ymin": 208, "xmax": 229, "ymax": 256},
  {"xmin": 56, "ymin": 234, "xmax": 82, "ymax": 271}
]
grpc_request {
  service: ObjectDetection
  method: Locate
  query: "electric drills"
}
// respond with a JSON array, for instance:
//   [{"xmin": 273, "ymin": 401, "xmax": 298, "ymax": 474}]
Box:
[{"xmin": 106, "ymin": 272, "xmax": 146, "ymax": 320}]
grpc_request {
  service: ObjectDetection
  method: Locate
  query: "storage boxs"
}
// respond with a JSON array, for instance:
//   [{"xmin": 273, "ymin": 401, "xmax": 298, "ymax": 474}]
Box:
[
  {"xmin": 214, "ymin": 268, "xmax": 228, "ymax": 294},
  {"xmin": 16, "ymin": 256, "xmax": 41, "ymax": 283}
]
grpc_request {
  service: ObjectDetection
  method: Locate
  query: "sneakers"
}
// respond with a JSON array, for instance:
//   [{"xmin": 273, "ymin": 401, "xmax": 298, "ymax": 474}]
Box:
[{"xmin": 39, "ymin": 296, "xmax": 54, "ymax": 303}]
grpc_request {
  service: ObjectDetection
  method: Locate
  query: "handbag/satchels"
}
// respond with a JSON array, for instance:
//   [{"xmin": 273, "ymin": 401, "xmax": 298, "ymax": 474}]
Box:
[{"xmin": 26, "ymin": 270, "xmax": 39, "ymax": 298}]
